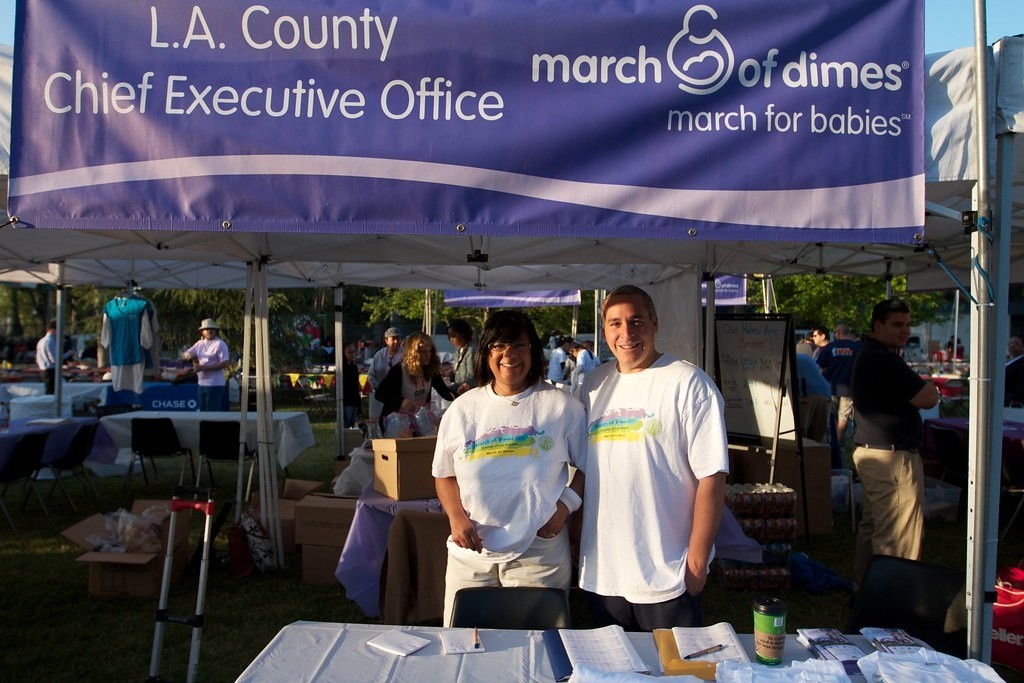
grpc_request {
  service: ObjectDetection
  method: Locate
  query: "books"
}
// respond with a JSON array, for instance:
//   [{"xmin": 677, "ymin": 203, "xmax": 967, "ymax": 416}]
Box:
[
  {"xmin": 366, "ymin": 628, "xmax": 431, "ymax": 656},
  {"xmin": 539, "ymin": 623, "xmax": 650, "ymax": 683},
  {"xmin": 671, "ymin": 622, "xmax": 752, "ymax": 667},
  {"xmin": 439, "ymin": 628, "xmax": 485, "ymax": 655}
]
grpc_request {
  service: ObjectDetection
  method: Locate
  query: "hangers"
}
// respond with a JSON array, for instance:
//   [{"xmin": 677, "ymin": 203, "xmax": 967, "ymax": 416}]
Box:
[{"xmin": 115, "ymin": 280, "xmax": 133, "ymax": 310}]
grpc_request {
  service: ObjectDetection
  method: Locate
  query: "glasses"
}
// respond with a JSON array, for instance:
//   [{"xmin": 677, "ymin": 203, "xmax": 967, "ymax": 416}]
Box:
[
  {"xmin": 890, "ymin": 299, "xmax": 909, "ymax": 309},
  {"xmin": 388, "ymin": 355, "xmax": 393, "ymax": 367},
  {"xmin": 487, "ymin": 340, "xmax": 532, "ymax": 353}
]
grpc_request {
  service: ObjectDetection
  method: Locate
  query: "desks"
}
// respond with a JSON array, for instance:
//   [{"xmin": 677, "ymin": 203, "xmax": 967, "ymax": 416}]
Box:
[
  {"xmin": 335, "ymin": 483, "xmax": 762, "ymax": 617},
  {"xmin": 98, "ymin": 412, "xmax": 315, "ymax": 501},
  {"xmin": 921, "ymin": 417, "xmax": 1024, "ymax": 540},
  {"xmin": 231, "ymin": 620, "xmax": 939, "ymax": 683},
  {"xmin": 0, "ymin": 418, "xmax": 117, "ymax": 520},
  {"xmin": 1, "ymin": 381, "xmax": 199, "ymax": 427},
  {"xmin": 726, "ymin": 433, "xmax": 834, "ymax": 543}
]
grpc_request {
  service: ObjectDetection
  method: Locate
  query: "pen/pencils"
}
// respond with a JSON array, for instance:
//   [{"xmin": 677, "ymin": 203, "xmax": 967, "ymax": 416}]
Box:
[
  {"xmin": 474, "ymin": 626, "xmax": 479, "ymax": 649},
  {"xmin": 684, "ymin": 644, "xmax": 723, "ymax": 659}
]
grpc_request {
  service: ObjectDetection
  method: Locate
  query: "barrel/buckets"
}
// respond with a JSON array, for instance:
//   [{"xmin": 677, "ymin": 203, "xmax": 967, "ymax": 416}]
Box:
[{"xmin": 215, "ymin": 526, "xmax": 253, "ymax": 579}]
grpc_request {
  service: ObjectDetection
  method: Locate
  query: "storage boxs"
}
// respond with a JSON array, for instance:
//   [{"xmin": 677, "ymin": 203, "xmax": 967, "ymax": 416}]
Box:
[
  {"xmin": 61, "ymin": 500, "xmax": 190, "ymax": 597},
  {"xmin": 250, "ymin": 428, "xmax": 438, "ymax": 585},
  {"xmin": 832, "ymin": 470, "xmax": 962, "ymax": 527}
]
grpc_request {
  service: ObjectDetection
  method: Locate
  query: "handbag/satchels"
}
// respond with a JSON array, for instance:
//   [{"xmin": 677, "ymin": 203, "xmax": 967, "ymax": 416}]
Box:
[
  {"xmin": 990, "ymin": 559, "xmax": 1023, "ymax": 676},
  {"xmin": 333, "ymin": 441, "xmax": 375, "ymax": 498}
]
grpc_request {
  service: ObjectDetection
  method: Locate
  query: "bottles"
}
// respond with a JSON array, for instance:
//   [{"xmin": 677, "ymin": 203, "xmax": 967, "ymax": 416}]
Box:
[{"xmin": 718, "ymin": 483, "xmax": 797, "ymax": 591}]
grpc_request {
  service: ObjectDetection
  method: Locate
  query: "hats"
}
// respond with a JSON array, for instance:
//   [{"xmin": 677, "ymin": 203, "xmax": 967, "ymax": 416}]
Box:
[
  {"xmin": 384, "ymin": 327, "xmax": 402, "ymax": 338},
  {"xmin": 198, "ymin": 319, "xmax": 220, "ymax": 330}
]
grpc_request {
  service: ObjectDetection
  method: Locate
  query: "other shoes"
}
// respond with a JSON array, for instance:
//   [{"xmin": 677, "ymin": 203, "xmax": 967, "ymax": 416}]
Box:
[{"xmin": 345, "ymin": 426, "xmax": 360, "ymax": 430}]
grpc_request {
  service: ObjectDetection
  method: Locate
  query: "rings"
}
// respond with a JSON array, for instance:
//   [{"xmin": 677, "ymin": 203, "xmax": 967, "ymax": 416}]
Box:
[{"xmin": 550, "ymin": 534, "xmax": 556, "ymax": 537}]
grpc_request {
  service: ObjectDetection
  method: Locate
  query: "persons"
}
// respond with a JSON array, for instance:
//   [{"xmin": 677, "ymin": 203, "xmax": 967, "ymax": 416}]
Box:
[
  {"xmin": 446, "ymin": 317, "xmax": 483, "ymax": 397},
  {"xmin": 430, "ymin": 308, "xmax": 588, "ymax": 630},
  {"xmin": 363, "ymin": 326, "xmax": 405, "ymax": 425},
  {"xmin": 74, "ymin": 322, "xmax": 85, "ymax": 334},
  {"xmin": 304, "ymin": 323, "xmax": 320, "ymax": 349},
  {"xmin": 35, "ymin": 316, "xmax": 77, "ymax": 395},
  {"xmin": 571, "ymin": 282, "xmax": 731, "ymax": 633},
  {"xmin": 182, "ymin": 318, "xmax": 231, "ymax": 412},
  {"xmin": 796, "ymin": 321, "xmax": 862, "ymax": 447},
  {"xmin": 62, "ymin": 334, "xmax": 74, "ymax": 365},
  {"xmin": 547, "ymin": 337, "xmax": 602, "ymax": 397},
  {"xmin": 374, "ymin": 331, "xmax": 471, "ymax": 440},
  {"xmin": 956, "ymin": 338, "xmax": 964, "ymax": 363},
  {"xmin": 0, "ymin": 339, "xmax": 28, "ymax": 364},
  {"xmin": 1004, "ymin": 331, "xmax": 1024, "ymax": 409},
  {"xmin": 81, "ymin": 341, "xmax": 97, "ymax": 367},
  {"xmin": 357, "ymin": 332, "xmax": 374, "ymax": 359},
  {"xmin": 852, "ymin": 299, "xmax": 938, "ymax": 598},
  {"xmin": 947, "ymin": 335, "xmax": 954, "ymax": 362},
  {"xmin": 342, "ymin": 343, "xmax": 362, "ymax": 429}
]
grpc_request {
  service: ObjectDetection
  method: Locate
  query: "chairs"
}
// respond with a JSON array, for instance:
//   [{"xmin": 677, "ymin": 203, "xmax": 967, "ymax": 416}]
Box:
[
  {"xmin": 34, "ymin": 422, "xmax": 103, "ymax": 520},
  {"xmin": 301, "ymin": 381, "xmax": 337, "ymax": 420},
  {"xmin": 121, "ymin": 419, "xmax": 194, "ymax": 501},
  {"xmin": 450, "ymin": 586, "xmax": 571, "ymax": 632},
  {"xmin": 0, "ymin": 428, "xmax": 60, "ymax": 531},
  {"xmin": 190, "ymin": 421, "xmax": 259, "ymax": 510}
]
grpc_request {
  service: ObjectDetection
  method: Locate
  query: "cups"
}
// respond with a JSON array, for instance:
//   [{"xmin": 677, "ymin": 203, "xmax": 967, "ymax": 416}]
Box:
[{"xmin": 752, "ymin": 596, "xmax": 789, "ymax": 666}]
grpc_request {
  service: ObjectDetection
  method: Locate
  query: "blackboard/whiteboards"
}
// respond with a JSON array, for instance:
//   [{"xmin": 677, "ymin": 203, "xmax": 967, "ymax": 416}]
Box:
[{"xmin": 715, "ymin": 314, "xmax": 803, "ymax": 453}]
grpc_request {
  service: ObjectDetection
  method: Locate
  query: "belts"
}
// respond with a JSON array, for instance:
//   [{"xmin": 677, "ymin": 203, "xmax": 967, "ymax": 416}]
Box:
[{"xmin": 859, "ymin": 441, "xmax": 915, "ymax": 450}]
